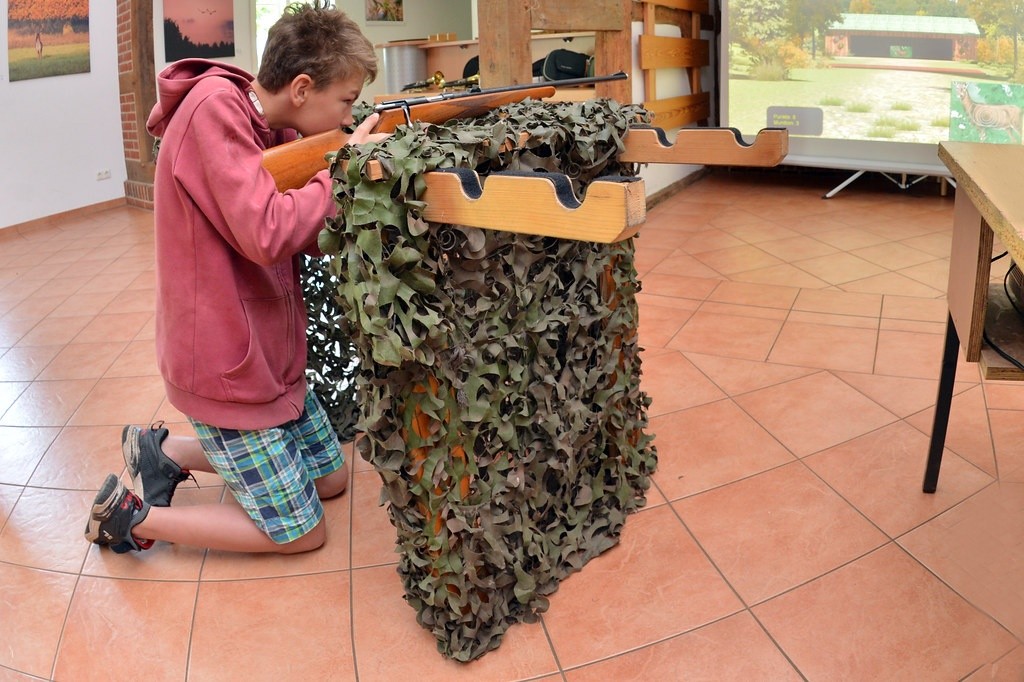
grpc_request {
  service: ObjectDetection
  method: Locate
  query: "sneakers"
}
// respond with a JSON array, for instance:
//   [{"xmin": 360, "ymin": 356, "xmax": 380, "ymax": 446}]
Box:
[
  {"xmin": 84, "ymin": 474, "xmax": 155, "ymax": 554},
  {"xmin": 121, "ymin": 421, "xmax": 200, "ymax": 507}
]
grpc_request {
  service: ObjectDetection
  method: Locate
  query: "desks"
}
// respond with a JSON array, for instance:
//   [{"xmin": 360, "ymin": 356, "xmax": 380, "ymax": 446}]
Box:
[{"xmin": 922, "ymin": 140, "xmax": 1024, "ymax": 491}]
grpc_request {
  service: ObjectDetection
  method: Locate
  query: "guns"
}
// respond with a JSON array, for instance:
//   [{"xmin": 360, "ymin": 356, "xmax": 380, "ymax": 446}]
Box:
[{"xmin": 259, "ymin": 69, "xmax": 629, "ymax": 195}]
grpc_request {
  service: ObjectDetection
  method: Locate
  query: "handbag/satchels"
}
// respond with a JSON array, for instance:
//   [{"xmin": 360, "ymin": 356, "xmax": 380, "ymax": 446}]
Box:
[
  {"xmin": 584, "ymin": 55, "xmax": 595, "ymax": 77},
  {"xmin": 543, "ymin": 48, "xmax": 590, "ymax": 81}
]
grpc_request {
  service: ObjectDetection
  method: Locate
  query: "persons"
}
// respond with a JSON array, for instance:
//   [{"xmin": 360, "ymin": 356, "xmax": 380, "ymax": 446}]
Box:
[{"xmin": 85, "ymin": 1, "xmax": 394, "ymax": 556}]
